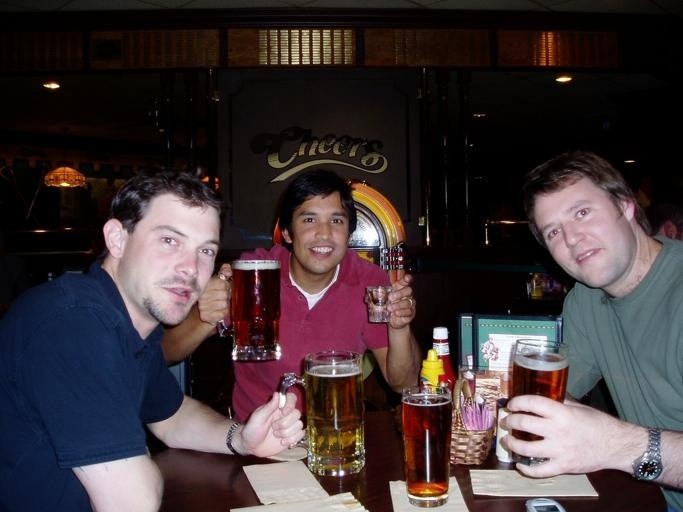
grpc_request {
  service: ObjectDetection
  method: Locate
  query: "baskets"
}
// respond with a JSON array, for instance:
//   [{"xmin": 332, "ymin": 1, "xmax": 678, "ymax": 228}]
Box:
[{"xmin": 447, "ymin": 379, "xmax": 498, "ymax": 469}]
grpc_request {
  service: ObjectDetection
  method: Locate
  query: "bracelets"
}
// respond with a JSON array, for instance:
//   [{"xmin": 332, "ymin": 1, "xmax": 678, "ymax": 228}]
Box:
[{"xmin": 226, "ymin": 420, "xmax": 242, "ymax": 456}]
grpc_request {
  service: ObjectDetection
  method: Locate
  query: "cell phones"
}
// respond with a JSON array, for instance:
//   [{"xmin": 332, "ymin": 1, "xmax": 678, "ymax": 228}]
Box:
[{"xmin": 526, "ymin": 499, "xmax": 566, "ymax": 512}]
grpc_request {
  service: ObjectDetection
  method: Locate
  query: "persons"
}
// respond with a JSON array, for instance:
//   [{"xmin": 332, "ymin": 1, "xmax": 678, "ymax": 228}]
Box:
[
  {"xmin": 162, "ymin": 167, "xmax": 424, "ymax": 423},
  {"xmin": 499, "ymin": 147, "xmax": 683, "ymax": 512},
  {"xmin": 0, "ymin": 169, "xmax": 307, "ymax": 511}
]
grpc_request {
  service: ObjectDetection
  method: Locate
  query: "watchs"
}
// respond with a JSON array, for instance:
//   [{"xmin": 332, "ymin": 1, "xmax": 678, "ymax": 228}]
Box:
[{"xmin": 633, "ymin": 426, "xmax": 664, "ymax": 482}]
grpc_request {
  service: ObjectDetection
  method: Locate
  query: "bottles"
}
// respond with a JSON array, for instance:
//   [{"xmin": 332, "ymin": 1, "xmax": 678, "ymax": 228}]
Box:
[
  {"xmin": 417, "ymin": 326, "xmax": 458, "ymax": 391},
  {"xmin": 527, "ymin": 272, "xmax": 543, "ymax": 297}
]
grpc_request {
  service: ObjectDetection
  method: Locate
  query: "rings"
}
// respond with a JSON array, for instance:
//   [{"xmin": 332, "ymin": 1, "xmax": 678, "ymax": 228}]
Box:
[{"xmin": 408, "ymin": 298, "xmax": 413, "ymax": 308}]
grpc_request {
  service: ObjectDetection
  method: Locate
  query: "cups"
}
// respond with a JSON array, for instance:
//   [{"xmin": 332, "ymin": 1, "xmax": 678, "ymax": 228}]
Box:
[
  {"xmin": 509, "ymin": 339, "xmax": 569, "ymax": 466},
  {"xmin": 279, "ymin": 350, "xmax": 366, "ymax": 478},
  {"xmin": 214, "ymin": 260, "xmax": 280, "ymax": 363},
  {"xmin": 402, "ymin": 386, "xmax": 453, "ymax": 507},
  {"xmin": 364, "ymin": 285, "xmax": 396, "ymax": 323}
]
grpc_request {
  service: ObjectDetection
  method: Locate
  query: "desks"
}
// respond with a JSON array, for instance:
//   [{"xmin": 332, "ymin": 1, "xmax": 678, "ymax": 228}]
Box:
[{"xmin": 140, "ymin": 406, "xmax": 668, "ymax": 512}]
